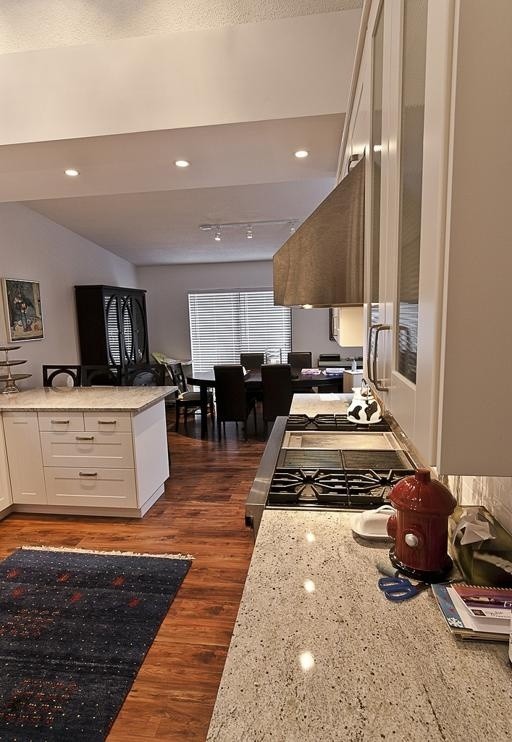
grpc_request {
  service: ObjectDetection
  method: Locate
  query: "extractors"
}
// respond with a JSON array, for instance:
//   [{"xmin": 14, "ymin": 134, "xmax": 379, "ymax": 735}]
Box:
[{"xmin": 273, "ymin": 153, "xmax": 363, "ymax": 307}]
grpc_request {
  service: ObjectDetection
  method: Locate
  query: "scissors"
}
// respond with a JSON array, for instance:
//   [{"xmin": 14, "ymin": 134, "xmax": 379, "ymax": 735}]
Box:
[{"xmin": 378, "ymin": 577, "xmax": 451, "ymax": 600}]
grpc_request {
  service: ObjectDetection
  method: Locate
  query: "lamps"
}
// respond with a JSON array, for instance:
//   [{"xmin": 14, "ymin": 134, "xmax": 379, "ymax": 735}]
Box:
[{"xmin": 199, "ymin": 218, "xmax": 305, "ymax": 241}]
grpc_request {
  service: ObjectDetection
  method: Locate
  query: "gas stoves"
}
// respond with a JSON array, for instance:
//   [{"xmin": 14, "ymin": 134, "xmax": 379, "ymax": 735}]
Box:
[{"xmin": 244, "ymin": 411, "xmax": 417, "ymax": 526}]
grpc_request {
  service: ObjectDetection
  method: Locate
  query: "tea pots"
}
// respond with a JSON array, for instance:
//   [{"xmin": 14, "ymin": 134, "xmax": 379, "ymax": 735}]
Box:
[{"xmin": 345, "ymin": 379, "xmax": 385, "ymax": 424}]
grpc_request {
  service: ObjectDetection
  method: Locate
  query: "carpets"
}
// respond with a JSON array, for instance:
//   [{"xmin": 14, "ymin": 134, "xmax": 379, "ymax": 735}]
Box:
[{"xmin": 1, "ymin": 546, "xmax": 192, "ymax": 742}]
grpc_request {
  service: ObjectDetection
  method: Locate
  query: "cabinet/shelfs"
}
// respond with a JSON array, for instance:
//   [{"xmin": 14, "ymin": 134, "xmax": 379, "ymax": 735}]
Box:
[
  {"xmin": 1, "ymin": 387, "xmax": 180, "ymax": 522},
  {"xmin": 329, "ymin": 0, "xmax": 512, "ymax": 477},
  {"xmin": 74, "ymin": 285, "xmax": 149, "ymax": 375}
]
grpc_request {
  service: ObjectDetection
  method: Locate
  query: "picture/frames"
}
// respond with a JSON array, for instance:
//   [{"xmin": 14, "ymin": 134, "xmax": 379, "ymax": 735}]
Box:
[{"xmin": 2, "ymin": 277, "xmax": 43, "ymax": 343}]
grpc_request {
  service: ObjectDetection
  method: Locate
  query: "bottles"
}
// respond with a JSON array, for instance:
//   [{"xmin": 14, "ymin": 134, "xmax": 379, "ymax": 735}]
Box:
[{"xmin": 351, "ymin": 360, "xmax": 356, "ymax": 372}]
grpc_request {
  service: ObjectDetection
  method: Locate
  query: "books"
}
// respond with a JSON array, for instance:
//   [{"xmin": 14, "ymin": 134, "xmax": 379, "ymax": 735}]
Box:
[{"xmin": 429, "ymin": 582, "xmax": 512, "ymax": 642}]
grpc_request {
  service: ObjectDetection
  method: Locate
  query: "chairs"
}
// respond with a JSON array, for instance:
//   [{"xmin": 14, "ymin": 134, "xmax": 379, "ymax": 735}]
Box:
[
  {"xmin": 82, "ymin": 365, "xmax": 121, "ymax": 386},
  {"xmin": 168, "ymin": 352, "xmax": 350, "ymax": 441},
  {"xmin": 42, "ymin": 365, "xmax": 81, "ymax": 387}
]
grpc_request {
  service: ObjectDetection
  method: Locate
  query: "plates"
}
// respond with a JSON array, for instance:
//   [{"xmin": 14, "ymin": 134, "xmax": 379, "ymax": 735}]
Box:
[
  {"xmin": 1, "ymin": 374, "xmax": 30, "ymax": 381},
  {"xmin": 0, "ymin": 346, "xmax": 22, "ymax": 352},
  {"xmin": 350, "ymin": 512, "xmax": 394, "ymax": 542},
  {"xmin": 1, "ymin": 359, "xmax": 27, "ymax": 367}
]
